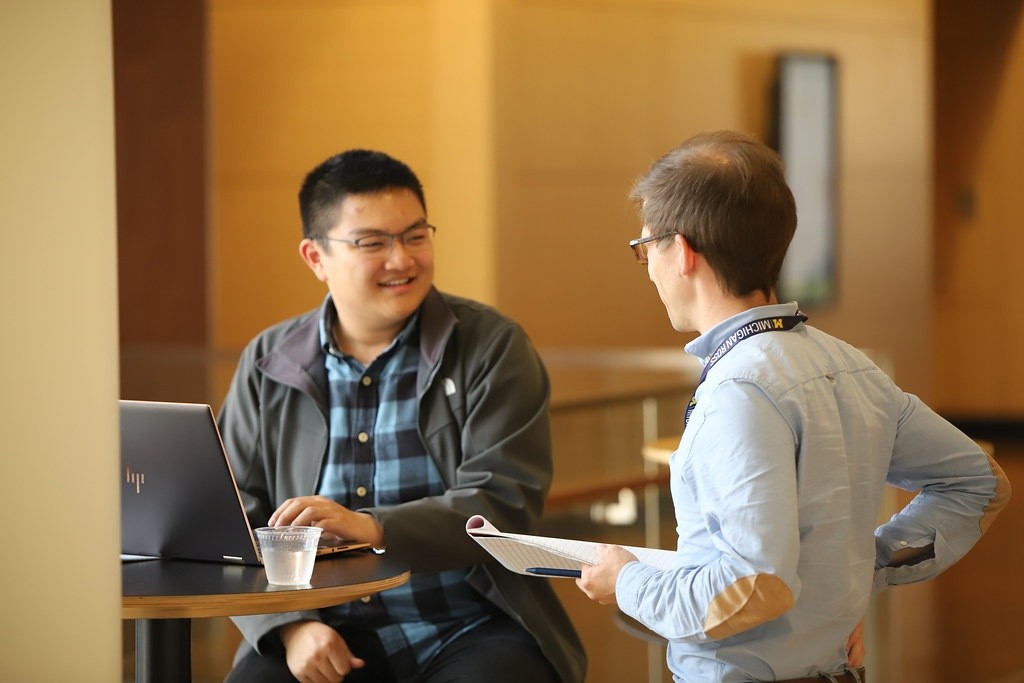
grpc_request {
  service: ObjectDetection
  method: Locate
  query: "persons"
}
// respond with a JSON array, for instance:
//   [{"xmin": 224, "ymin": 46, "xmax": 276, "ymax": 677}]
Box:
[
  {"xmin": 202, "ymin": 148, "xmax": 588, "ymax": 683},
  {"xmin": 574, "ymin": 132, "xmax": 1016, "ymax": 682}
]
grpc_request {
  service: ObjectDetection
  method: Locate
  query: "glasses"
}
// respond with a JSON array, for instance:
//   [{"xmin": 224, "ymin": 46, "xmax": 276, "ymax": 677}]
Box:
[
  {"xmin": 629, "ymin": 230, "xmax": 701, "ymax": 268},
  {"xmin": 310, "ymin": 223, "xmax": 437, "ymax": 260}
]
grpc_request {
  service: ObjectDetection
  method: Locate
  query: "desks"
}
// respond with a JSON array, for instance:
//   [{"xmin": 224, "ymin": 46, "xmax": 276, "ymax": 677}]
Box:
[
  {"xmin": 491, "ymin": 351, "xmax": 708, "ymax": 549},
  {"xmin": 120, "ymin": 547, "xmax": 413, "ymax": 683}
]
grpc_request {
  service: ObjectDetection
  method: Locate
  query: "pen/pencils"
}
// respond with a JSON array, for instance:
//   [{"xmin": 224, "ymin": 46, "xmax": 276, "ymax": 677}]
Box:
[{"xmin": 522, "ymin": 566, "xmax": 584, "ymax": 578}]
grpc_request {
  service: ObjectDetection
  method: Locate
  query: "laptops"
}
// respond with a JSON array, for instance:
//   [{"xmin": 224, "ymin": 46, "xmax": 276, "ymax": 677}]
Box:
[{"xmin": 120, "ymin": 400, "xmax": 372, "ymax": 569}]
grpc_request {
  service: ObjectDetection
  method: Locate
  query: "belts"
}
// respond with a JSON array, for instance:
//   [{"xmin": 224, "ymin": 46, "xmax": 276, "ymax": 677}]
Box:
[{"xmin": 742, "ymin": 668, "xmax": 866, "ymax": 683}]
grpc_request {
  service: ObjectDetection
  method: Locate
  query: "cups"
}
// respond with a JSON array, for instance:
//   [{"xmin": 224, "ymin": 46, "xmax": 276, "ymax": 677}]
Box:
[{"xmin": 256, "ymin": 524, "xmax": 323, "ymax": 587}]
